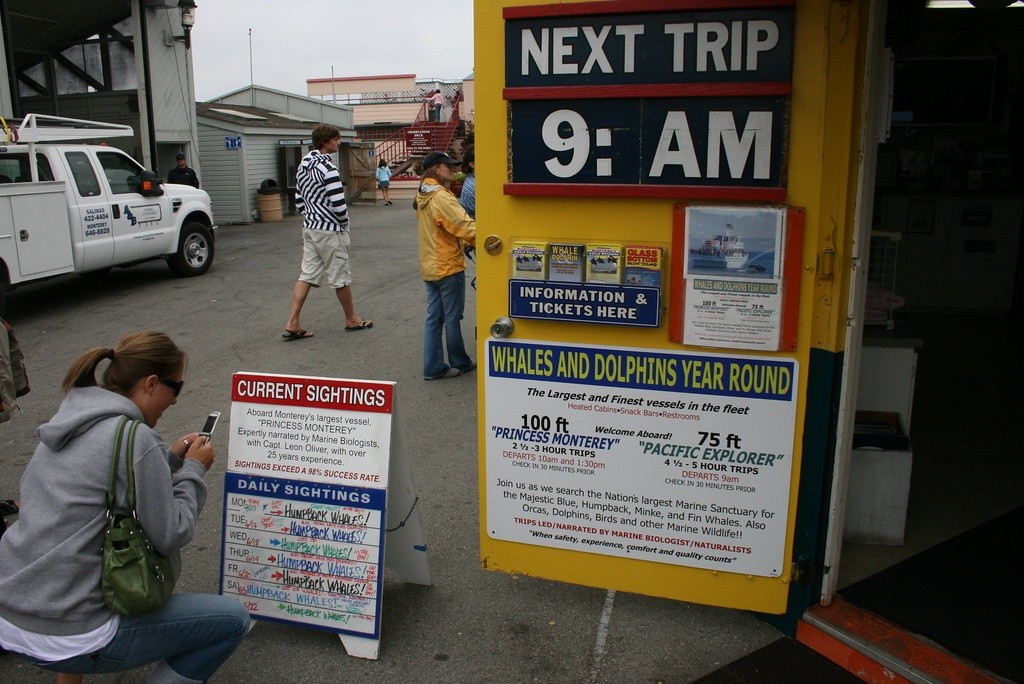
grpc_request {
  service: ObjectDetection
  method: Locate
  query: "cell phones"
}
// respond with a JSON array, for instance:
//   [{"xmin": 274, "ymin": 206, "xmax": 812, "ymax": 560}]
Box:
[{"xmin": 198, "ymin": 411, "xmax": 220, "ymax": 451}]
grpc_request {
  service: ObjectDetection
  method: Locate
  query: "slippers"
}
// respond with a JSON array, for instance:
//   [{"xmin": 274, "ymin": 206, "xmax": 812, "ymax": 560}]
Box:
[
  {"xmin": 345, "ymin": 319, "xmax": 373, "ymax": 330},
  {"xmin": 281, "ymin": 330, "xmax": 314, "ymax": 338}
]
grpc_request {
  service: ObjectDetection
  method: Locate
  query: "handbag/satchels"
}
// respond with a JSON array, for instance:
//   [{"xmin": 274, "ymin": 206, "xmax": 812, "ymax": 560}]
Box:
[{"xmin": 99, "ymin": 417, "xmax": 180, "ymax": 619}]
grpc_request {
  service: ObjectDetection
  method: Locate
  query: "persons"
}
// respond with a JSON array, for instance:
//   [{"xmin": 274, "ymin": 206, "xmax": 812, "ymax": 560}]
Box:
[
  {"xmin": 461, "ymin": 143, "xmax": 475, "ymax": 220},
  {"xmin": 0, "ymin": 307, "xmax": 32, "ymax": 540},
  {"xmin": 167, "ymin": 153, "xmax": 199, "ymax": 189},
  {"xmin": 284, "ymin": 123, "xmax": 372, "ymax": 337},
  {"xmin": 425, "ymin": 89, "xmax": 444, "ymax": 122},
  {"xmin": 375, "ymin": 159, "xmax": 393, "ymax": 205},
  {"xmin": 0, "ymin": 331, "xmax": 252, "ymax": 684},
  {"xmin": 416, "ymin": 149, "xmax": 478, "ymax": 380}
]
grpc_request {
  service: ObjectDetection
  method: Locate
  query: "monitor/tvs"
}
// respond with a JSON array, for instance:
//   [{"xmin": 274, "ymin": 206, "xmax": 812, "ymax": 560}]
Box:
[{"xmin": 890, "ymin": 56, "xmax": 998, "ymax": 126}]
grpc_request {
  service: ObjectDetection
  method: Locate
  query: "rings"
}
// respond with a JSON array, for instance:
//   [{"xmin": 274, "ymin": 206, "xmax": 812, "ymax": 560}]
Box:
[{"xmin": 183, "ymin": 439, "xmax": 190, "ymax": 447}]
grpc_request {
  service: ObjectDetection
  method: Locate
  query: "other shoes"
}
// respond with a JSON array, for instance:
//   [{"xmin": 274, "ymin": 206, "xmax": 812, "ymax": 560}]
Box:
[
  {"xmin": 461, "ymin": 360, "xmax": 477, "ymax": 372},
  {"xmin": 385, "ymin": 200, "xmax": 391, "ymax": 206},
  {"xmin": 423, "ymin": 367, "xmax": 461, "ymax": 381}
]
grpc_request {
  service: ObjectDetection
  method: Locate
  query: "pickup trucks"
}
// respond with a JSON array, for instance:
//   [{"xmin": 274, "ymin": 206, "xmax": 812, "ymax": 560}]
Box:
[{"xmin": 0, "ymin": 113, "xmax": 216, "ymax": 293}]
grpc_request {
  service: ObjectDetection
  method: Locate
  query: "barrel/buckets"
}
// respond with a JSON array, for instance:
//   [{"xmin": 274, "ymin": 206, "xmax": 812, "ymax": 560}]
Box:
[{"xmin": 255, "ymin": 192, "xmax": 283, "ymax": 222}]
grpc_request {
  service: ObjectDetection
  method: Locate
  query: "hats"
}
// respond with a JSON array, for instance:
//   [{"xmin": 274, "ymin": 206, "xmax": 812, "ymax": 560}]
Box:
[
  {"xmin": 176, "ymin": 154, "xmax": 185, "ymax": 161},
  {"xmin": 423, "ymin": 150, "xmax": 463, "ymax": 170}
]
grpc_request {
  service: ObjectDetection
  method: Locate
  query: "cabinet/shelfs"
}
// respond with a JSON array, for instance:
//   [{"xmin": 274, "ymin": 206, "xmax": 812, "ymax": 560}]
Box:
[{"xmin": 871, "ymin": 194, "xmax": 1022, "ymax": 319}]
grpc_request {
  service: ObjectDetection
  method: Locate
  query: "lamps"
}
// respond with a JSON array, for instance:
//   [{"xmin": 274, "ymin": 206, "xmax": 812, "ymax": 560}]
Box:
[{"xmin": 162, "ymin": 0, "xmax": 197, "ymax": 50}]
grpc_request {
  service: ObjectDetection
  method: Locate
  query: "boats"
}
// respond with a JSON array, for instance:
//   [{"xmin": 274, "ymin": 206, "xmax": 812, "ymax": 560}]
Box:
[{"xmin": 688, "ymin": 215, "xmax": 748, "ymax": 269}]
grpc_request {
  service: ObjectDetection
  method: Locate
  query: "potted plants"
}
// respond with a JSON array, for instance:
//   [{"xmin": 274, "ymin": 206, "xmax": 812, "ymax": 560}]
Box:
[{"xmin": 256, "ymin": 178, "xmax": 283, "ymax": 221}]
